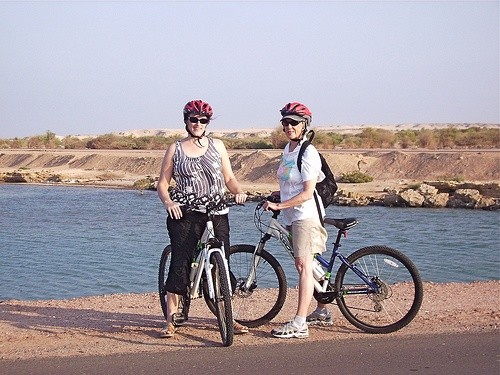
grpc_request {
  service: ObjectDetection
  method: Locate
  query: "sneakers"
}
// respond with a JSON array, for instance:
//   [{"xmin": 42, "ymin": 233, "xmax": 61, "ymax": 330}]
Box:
[
  {"xmin": 305, "ymin": 312, "xmax": 333, "ymax": 327},
  {"xmin": 271, "ymin": 320, "xmax": 309, "ymax": 339}
]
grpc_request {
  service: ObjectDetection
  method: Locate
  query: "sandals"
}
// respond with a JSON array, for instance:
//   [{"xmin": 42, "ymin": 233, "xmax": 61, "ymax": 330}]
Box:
[
  {"xmin": 233, "ymin": 319, "xmax": 248, "ymax": 334},
  {"xmin": 160, "ymin": 323, "xmax": 175, "ymax": 337}
]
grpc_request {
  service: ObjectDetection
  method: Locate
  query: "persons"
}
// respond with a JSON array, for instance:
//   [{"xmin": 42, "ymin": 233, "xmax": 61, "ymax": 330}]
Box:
[
  {"xmin": 263, "ymin": 102, "xmax": 333, "ymax": 339},
  {"xmin": 157, "ymin": 100, "xmax": 248, "ymax": 337}
]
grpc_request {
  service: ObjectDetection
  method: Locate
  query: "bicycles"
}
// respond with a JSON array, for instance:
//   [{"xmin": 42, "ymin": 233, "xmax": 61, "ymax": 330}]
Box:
[
  {"xmin": 158, "ymin": 197, "xmax": 252, "ymax": 347},
  {"xmin": 202, "ymin": 195, "xmax": 423, "ymax": 334}
]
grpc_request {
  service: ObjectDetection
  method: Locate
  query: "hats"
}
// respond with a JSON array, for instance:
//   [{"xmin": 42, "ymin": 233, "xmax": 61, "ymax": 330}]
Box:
[{"xmin": 279, "ymin": 115, "xmax": 305, "ymax": 122}]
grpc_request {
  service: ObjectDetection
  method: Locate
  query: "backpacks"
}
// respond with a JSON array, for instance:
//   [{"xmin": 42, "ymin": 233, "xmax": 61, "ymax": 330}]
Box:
[{"xmin": 297, "ymin": 130, "xmax": 338, "ymax": 209}]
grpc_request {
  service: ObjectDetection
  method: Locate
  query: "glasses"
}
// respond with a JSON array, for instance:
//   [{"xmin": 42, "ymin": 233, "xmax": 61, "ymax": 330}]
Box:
[
  {"xmin": 281, "ymin": 121, "xmax": 302, "ymax": 126},
  {"xmin": 186, "ymin": 116, "xmax": 209, "ymax": 124}
]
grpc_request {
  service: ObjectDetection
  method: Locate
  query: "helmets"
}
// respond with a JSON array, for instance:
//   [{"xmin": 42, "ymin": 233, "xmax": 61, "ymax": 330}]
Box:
[
  {"xmin": 280, "ymin": 102, "xmax": 313, "ymax": 126},
  {"xmin": 183, "ymin": 99, "xmax": 213, "ymax": 116}
]
